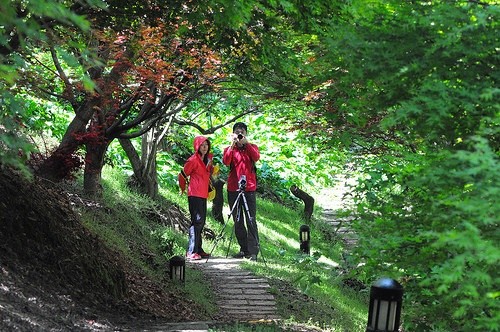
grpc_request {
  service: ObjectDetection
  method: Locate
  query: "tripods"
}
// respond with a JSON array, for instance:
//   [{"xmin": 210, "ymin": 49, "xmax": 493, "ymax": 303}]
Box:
[{"xmin": 205, "ymin": 188, "xmax": 267, "ymax": 267}]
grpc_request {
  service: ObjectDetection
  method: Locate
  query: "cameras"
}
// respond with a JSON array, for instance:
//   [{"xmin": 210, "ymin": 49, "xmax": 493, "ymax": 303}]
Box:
[
  {"xmin": 237, "ymin": 133, "xmax": 243, "ymax": 139},
  {"xmin": 238, "ymin": 175, "xmax": 247, "ymax": 188}
]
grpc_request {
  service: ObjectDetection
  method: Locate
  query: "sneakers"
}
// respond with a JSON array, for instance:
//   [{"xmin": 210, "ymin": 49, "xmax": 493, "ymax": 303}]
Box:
[
  {"xmin": 186, "ymin": 253, "xmax": 201, "ymax": 259},
  {"xmin": 233, "ymin": 252, "xmax": 249, "ymax": 258},
  {"xmin": 251, "ymin": 254, "xmax": 257, "ymax": 261},
  {"xmin": 199, "ymin": 252, "xmax": 210, "ymax": 257}
]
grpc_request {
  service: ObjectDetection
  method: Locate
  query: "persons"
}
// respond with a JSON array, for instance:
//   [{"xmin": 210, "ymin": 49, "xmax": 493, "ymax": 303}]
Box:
[
  {"xmin": 178, "ymin": 136, "xmax": 213, "ymax": 260},
  {"xmin": 223, "ymin": 123, "xmax": 261, "ymax": 260}
]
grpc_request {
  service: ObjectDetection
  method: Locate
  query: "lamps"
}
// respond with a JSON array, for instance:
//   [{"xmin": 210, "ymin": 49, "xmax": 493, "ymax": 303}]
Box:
[
  {"xmin": 299, "ymin": 225, "xmax": 310, "ymax": 254},
  {"xmin": 366, "ymin": 278, "xmax": 403, "ymax": 332}
]
